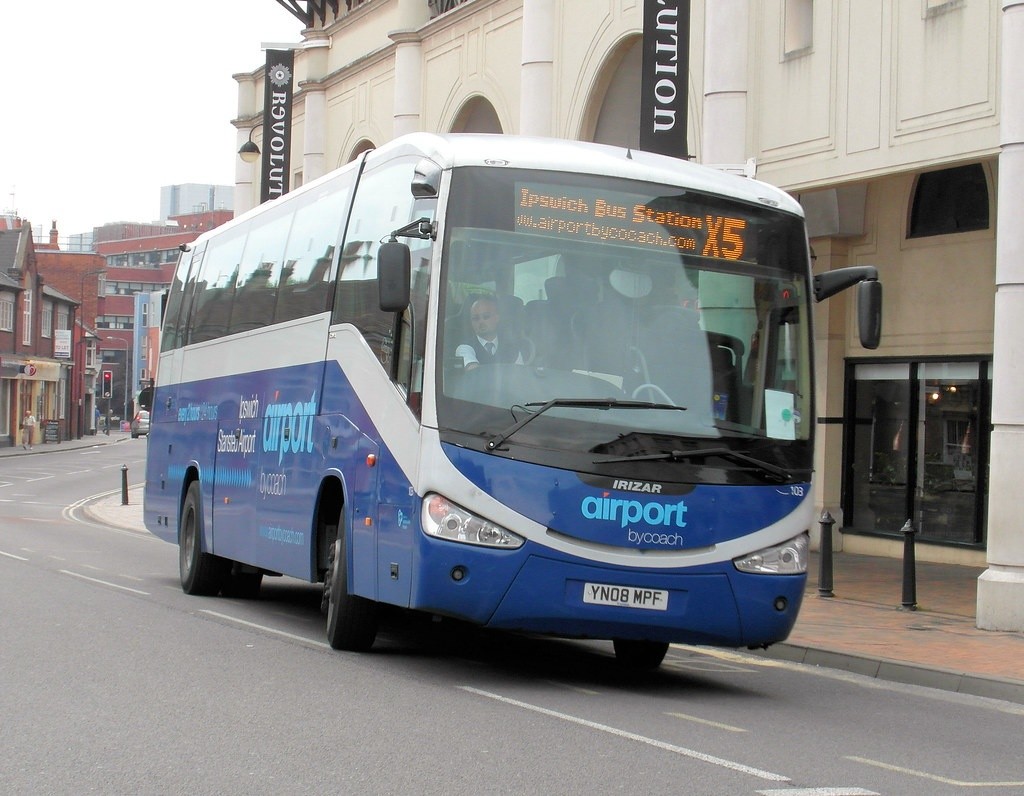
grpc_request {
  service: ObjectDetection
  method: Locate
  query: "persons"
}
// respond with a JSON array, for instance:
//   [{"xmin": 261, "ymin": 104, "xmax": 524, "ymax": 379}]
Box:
[
  {"xmin": 455, "ymin": 300, "xmax": 524, "ymax": 369},
  {"xmin": 22, "ymin": 410, "xmax": 36, "ymax": 449},
  {"xmin": 95, "ymin": 405, "xmax": 100, "ymax": 433},
  {"xmin": 103, "ymin": 409, "xmax": 113, "ymax": 433}
]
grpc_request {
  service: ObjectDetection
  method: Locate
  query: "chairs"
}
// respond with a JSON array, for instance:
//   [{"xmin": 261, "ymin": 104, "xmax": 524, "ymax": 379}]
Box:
[
  {"xmin": 705, "ymin": 329, "xmax": 746, "ymax": 424},
  {"xmin": 441, "ymin": 288, "xmax": 524, "ymax": 367}
]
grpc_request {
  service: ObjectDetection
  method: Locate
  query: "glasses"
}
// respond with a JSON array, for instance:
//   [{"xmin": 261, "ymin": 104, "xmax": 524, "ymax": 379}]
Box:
[{"xmin": 470, "ymin": 312, "xmax": 498, "ymax": 322}]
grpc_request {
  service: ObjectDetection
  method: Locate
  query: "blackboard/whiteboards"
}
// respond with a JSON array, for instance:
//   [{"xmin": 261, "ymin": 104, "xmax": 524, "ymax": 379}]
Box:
[{"xmin": 45, "ymin": 420, "xmax": 61, "ymax": 441}]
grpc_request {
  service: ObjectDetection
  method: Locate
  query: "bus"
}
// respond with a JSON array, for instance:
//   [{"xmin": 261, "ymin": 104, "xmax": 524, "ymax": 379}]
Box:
[{"xmin": 142, "ymin": 131, "xmax": 883, "ymax": 689}]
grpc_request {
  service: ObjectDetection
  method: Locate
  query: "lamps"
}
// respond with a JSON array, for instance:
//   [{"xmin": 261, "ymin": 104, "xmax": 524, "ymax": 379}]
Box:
[
  {"xmin": 238, "ymin": 124, "xmax": 264, "ymax": 162},
  {"xmin": 961, "ymin": 422, "xmax": 973, "ymax": 457},
  {"xmin": 892, "ymin": 420, "xmax": 905, "ymax": 453}
]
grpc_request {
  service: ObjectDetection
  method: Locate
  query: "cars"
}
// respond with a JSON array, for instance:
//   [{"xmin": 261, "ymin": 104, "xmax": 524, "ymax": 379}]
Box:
[{"xmin": 130, "ymin": 410, "xmax": 150, "ymax": 438}]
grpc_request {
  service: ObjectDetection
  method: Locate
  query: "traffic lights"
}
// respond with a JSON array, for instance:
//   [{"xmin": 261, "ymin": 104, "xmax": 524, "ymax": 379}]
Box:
[{"xmin": 102, "ymin": 371, "xmax": 112, "ymax": 398}]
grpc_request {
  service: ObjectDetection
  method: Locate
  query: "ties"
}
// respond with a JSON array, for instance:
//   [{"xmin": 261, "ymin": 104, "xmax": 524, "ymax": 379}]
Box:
[{"xmin": 485, "ymin": 340, "xmax": 492, "ymax": 355}]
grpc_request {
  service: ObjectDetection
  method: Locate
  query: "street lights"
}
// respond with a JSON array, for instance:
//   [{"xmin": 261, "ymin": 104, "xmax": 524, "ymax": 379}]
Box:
[{"xmin": 107, "ymin": 337, "xmax": 129, "ymax": 421}]
